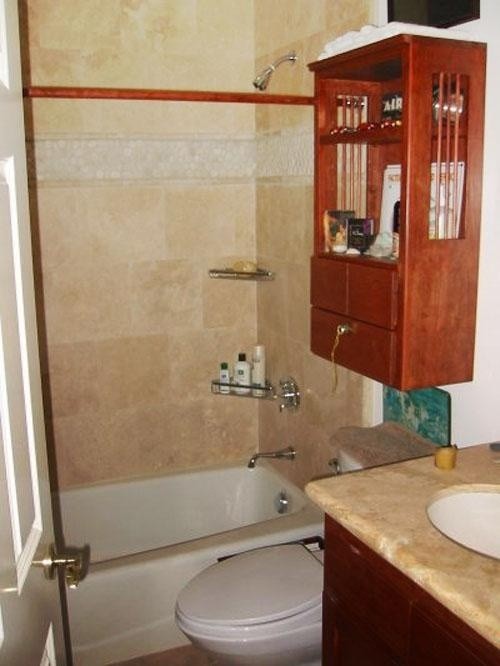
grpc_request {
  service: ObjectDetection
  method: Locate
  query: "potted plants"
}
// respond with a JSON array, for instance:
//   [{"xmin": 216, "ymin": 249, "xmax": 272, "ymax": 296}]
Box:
[{"xmin": 388, "ymin": 0, "xmax": 480, "ymax": 29}]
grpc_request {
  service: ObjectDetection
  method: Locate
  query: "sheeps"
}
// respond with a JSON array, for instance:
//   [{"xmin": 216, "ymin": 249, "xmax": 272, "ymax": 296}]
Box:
[{"xmin": 247, "ymin": 447, "xmax": 293, "ymax": 469}]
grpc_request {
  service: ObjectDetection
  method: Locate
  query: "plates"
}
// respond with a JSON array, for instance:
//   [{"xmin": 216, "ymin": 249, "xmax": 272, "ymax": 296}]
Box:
[
  {"xmin": 306, "ymin": 33, "xmax": 487, "ymax": 392},
  {"xmin": 322, "ymin": 515, "xmax": 500, "ymax": 665}
]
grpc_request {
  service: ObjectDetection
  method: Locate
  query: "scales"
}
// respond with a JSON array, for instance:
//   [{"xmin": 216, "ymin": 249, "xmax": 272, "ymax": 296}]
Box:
[{"xmin": 59, "ymin": 459, "xmax": 326, "ymax": 665}]
